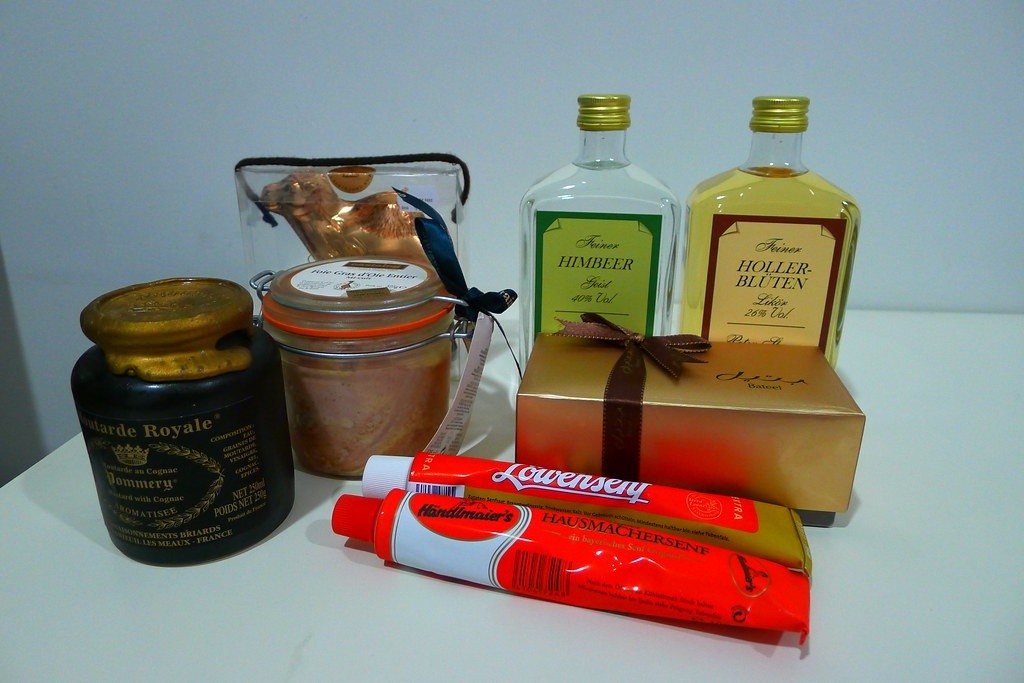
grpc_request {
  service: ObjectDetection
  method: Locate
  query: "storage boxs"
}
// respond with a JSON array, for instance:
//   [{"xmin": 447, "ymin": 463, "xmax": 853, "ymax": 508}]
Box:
[{"xmin": 513, "ymin": 332, "xmax": 866, "ymax": 514}]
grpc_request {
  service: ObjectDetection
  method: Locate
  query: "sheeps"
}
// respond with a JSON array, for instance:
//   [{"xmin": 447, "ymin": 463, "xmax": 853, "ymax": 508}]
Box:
[{"xmin": 261, "ymin": 169, "xmax": 434, "ymax": 273}]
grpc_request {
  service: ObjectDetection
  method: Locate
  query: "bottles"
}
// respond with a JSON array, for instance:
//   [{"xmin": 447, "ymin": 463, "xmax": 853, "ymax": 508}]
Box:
[
  {"xmin": 679, "ymin": 96, "xmax": 863, "ymax": 370},
  {"xmin": 520, "ymin": 94, "xmax": 681, "ymax": 389},
  {"xmin": 252, "ymin": 255, "xmax": 479, "ymax": 480},
  {"xmin": 71, "ymin": 276, "xmax": 295, "ymax": 568}
]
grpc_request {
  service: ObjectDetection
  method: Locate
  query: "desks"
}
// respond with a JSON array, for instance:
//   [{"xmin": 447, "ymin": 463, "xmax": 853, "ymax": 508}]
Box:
[{"xmin": 2, "ymin": 313, "xmax": 1024, "ymax": 683}]
